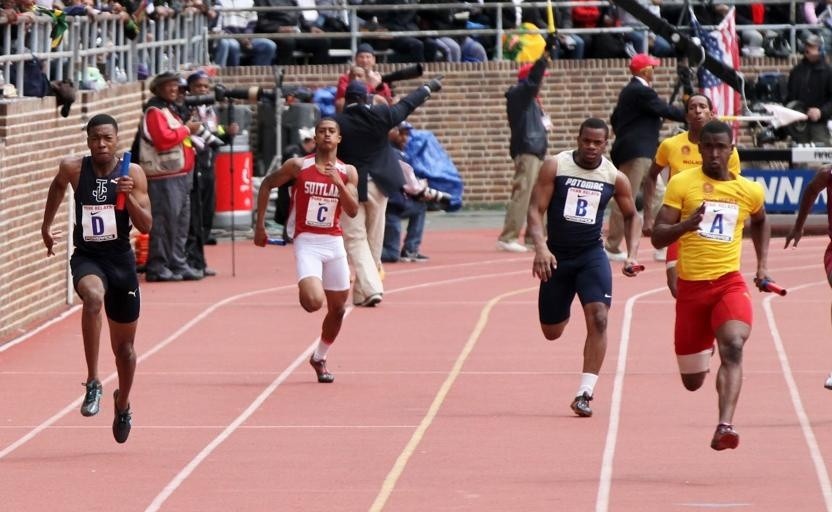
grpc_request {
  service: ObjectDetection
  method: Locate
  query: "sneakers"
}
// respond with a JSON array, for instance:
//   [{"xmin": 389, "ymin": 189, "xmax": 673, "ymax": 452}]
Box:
[
  {"xmin": 497, "ymin": 239, "xmax": 527, "ymax": 253},
  {"xmin": 711, "ymin": 423, "xmax": 738, "ymax": 450},
  {"xmin": 571, "ymin": 390, "xmax": 593, "ymax": 416},
  {"xmin": 113, "ymin": 389, "xmax": 132, "ymax": 443},
  {"xmin": 401, "ymin": 247, "xmax": 429, "ymax": 262},
  {"xmin": 603, "ymin": 247, "xmax": 628, "ymax": 261},
  {"xmin": 310, "ymin": 353, "xmax": 334, "ymax": 383},
  {"xmin": 81, "ymin": 380, "xmax": 102, "ymax": 416}
]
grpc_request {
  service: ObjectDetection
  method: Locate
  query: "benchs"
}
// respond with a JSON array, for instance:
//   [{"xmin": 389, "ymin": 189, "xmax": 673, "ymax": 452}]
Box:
[{"xmin": 208, "ymin": 44, "xmax": 395, "ymax": 63}]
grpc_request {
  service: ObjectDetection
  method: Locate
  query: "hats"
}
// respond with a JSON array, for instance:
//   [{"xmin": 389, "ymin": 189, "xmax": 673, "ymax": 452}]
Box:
[
  {"xmin": 631, "ymin": 55, "xmax": 659, "ymax": 72},
  {"xmin": 150, "ymin": 71, "xmax": 208, "ymax": 92},
  {"xmin": 356, "ymin": 43, "xmax": 373, "ymax": 54},
  {"xmin": 518, "ymin": 65, "xmax": 548, "ymax": 79},
  {"xmin": 347, "ymin": 81, "xmax": 368, "ymax": 95}
]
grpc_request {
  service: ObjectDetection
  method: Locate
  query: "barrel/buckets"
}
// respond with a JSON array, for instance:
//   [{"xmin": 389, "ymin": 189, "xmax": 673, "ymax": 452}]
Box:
[{"xmin": 209, "ymin": 143, "xmax": 257, "ymax": 231}]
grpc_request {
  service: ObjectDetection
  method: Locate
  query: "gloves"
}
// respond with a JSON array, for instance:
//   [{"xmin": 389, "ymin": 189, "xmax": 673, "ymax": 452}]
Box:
[{"xmin": 425, "ymin": 76, "xmax": 444, "ymax": 91}]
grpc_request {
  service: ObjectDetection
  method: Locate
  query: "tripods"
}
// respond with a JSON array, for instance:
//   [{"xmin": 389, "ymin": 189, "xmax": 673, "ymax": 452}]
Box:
[{"xmin": 662, "ymin": 77, "xmax": 696, "ymax": 131}]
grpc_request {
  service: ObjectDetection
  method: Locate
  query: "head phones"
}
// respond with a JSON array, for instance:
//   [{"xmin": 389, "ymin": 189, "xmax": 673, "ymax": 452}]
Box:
[{"xmin": 819, "ymin": 35, "xmax": 826, "ymax": 58}]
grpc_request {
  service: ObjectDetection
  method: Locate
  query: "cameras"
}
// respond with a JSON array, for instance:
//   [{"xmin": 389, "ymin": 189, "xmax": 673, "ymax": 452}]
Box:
[
  {"xmin": 215, "ymin": 83, "xmax": 264, "ymax": 103},
  {"xmin": 382, "ymin": 63, "xmax": 423, "ymax": 83},
  {"xmin": 195, "ymin": 124, "xmax": 225, "ymax": 152},
  {"xmin": 184, "ymin": 94, "xmax": 215, "ymax": 108}
]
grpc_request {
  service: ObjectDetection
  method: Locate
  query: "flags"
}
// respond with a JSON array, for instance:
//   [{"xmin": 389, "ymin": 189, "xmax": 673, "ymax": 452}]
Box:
[{"xmin": 690, "ymin": 8, "xmax": 741, "ymax": 146}]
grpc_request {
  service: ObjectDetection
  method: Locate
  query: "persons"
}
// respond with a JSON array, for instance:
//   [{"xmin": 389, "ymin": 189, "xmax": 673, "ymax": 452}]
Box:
[
  {"xmin": 525, "ymin": 121, "xmax": 643, "ymax": 415},
  {"xmin": 1, "ymin": 1, "xmax": 832, "ymax": 307},
  {"xmin": 40, "ymin": 112, "xmax": 152, "ymax": 446},
  {"xmin": 783, "ymin": 163, "xmax": 832, "ymax": 390},
  {"xmin": 253, "ymin": 118, "xmax": 360, "ymax": 382},
  {"xmin": 653, "ymin": 122, "xmax": 774, "ymax": 451}
]
